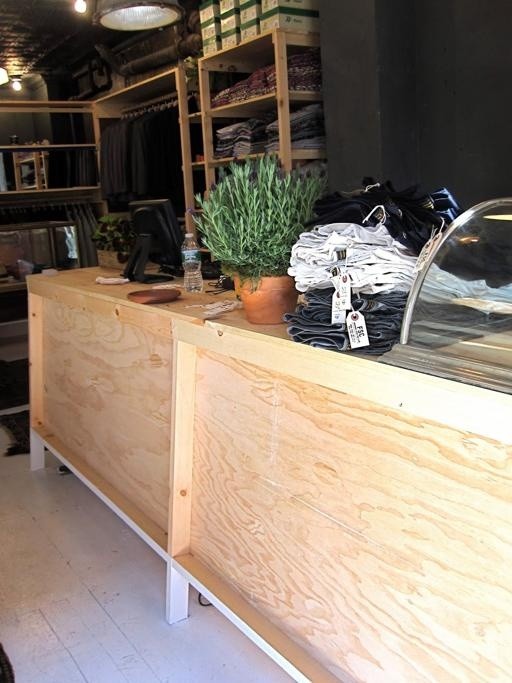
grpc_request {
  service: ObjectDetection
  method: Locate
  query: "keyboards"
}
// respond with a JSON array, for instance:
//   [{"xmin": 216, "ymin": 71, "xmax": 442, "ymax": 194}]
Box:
[{"xmin": 159, "ymin": 264, "xmax": 220, "ymax": 278}]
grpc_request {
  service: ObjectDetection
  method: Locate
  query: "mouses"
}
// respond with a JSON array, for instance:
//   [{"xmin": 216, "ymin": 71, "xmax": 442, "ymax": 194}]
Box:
[{"xmin": 223, "ymin": 277, "xmax": 232, "ymax": 288}]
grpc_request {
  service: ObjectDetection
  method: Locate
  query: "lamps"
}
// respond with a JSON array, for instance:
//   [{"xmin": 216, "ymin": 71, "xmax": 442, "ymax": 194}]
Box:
[{"xmin": 91, "ymin": 1, "xmax": 185, "ymax": 32}]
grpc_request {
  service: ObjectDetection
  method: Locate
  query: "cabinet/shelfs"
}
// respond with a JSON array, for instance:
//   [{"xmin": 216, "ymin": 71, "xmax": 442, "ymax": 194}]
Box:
[
  {"xmin": 198, "ymin": 29, "xmax": 326, "ymax": 206},
  {"xmin": 25, "ymin": 268, "xmax": 512, "ymax": 683}
]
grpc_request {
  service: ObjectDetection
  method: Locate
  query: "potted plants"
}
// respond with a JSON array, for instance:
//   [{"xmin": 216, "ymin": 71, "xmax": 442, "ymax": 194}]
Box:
[{"xmin": 192, "ymin": 156, "xmax": 320, "ymax": 325}]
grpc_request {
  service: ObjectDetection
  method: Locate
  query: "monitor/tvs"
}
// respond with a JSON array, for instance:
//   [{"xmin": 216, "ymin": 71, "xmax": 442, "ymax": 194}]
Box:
[{"xmin": 121, "ymin": 199, "xmax": 184, "ymax": 284}]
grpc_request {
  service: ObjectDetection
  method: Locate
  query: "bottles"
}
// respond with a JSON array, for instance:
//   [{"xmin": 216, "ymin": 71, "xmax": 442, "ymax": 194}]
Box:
[{"xmin": 180, "ymin": 233, "xmax": 203, "ymax": 293}]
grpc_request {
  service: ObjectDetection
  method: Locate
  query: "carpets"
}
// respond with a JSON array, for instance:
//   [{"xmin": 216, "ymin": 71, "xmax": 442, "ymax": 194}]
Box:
[{"xmin": 0, "ymin": 410, "xmax": 47, "ymax": 458}]
